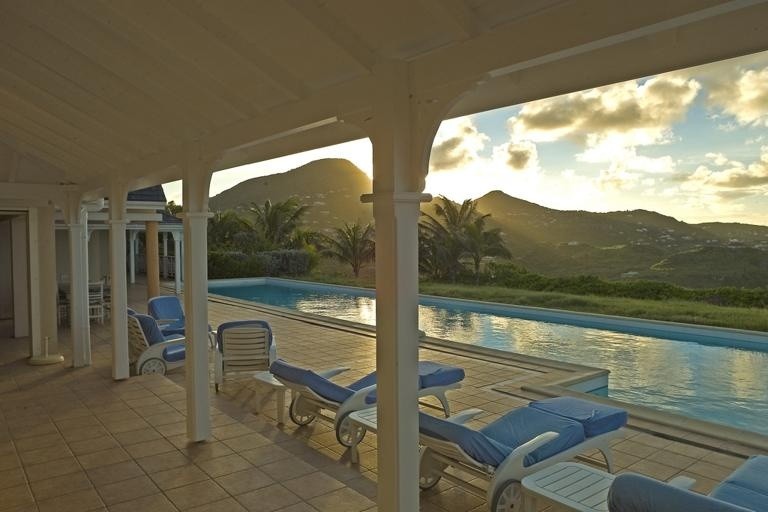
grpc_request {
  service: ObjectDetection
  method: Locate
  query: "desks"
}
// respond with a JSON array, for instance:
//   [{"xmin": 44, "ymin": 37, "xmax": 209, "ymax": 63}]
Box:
[
  {"xmin": 253, "ymin": 370, "xmax": 293, "ymax": 425},
  {"xmin": 346, "ymin": 407, "xmax": 378, "ymax": 464},
  {"xmin": 518, "ymin": 460, "xmax": 617, "ymax": 512}
]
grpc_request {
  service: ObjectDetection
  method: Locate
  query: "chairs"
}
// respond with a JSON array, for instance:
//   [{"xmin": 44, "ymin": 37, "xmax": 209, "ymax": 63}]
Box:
[
  {"xmin": 58, "ymin": 280, "xmax": 216, "ymax": 382},
  {"xmin": 599, "ymin": 452, "xmax": 766, "ymax": 512},
  {"xmin": 266, "ymin": 358, "xmax": 467, "ymax": 448},
  {"xmin": 418, "ymin": 395, "xmax": 628, "ymax": 512},
  {"xmin": 212, "ymin": 318, "xmax": 279, "ymax": 393}
]
